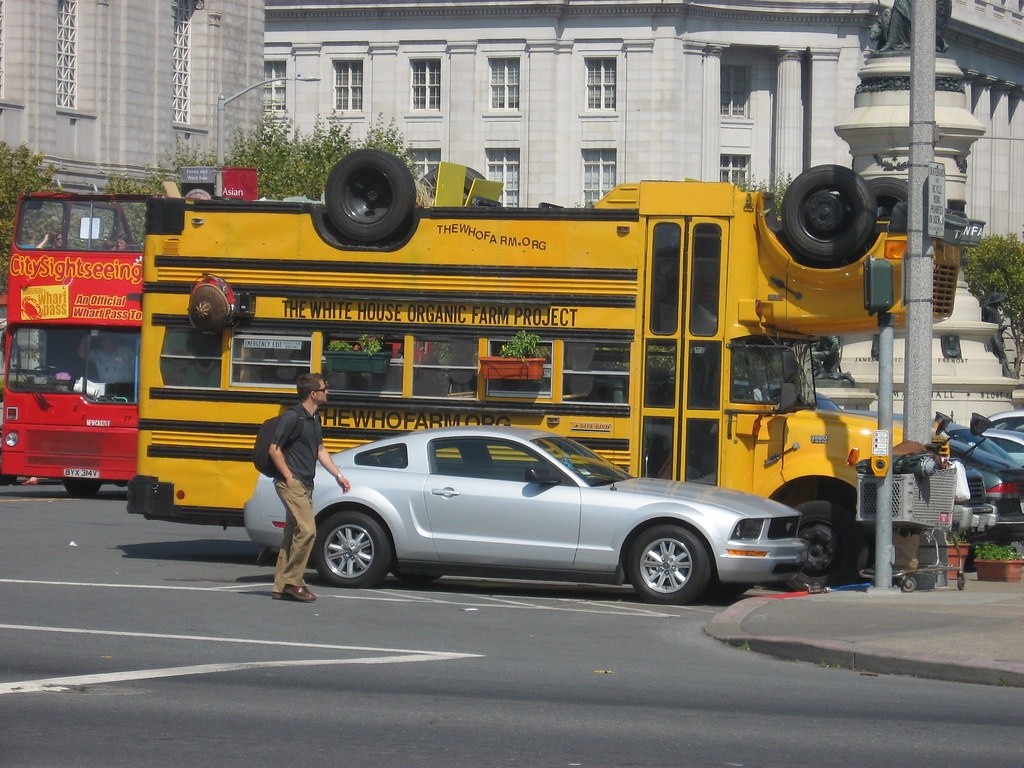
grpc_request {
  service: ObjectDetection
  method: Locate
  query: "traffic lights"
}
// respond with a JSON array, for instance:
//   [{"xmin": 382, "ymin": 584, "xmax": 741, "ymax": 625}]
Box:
[{"xmin": 864, "ymin": 256, "xmax": 893, "ymax": 316}]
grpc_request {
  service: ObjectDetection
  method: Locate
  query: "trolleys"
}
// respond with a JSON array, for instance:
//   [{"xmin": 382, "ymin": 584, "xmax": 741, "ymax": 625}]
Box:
[{"xmin": 856, "ymin": 469, "xmax": 963, "ymax": 585}]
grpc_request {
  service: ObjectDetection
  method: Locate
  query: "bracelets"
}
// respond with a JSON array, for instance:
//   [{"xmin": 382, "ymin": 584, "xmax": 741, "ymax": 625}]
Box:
[{"xmin": 335, "ymin": 473, "xmax": 342, "ymax": 480}]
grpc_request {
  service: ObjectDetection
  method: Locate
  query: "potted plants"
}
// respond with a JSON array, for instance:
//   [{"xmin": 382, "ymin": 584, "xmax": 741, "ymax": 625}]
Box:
[
  {"xmin": 948, "ymin": 532, "xmax": 970, "ymax": 579},
  {"xmin": 973, "ymin": 542, "xmax": 1024, "ymax": 583},
  {"xmin": 479, "ymin": 330, "xmax": 549, "ymax": 380},
  {"xmin": 322, "ymin": 333, "xmax": 391, "ymax": 373}
]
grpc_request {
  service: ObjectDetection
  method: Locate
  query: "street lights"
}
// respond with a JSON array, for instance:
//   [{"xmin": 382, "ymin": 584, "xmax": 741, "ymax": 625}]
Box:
[{"xmin": 217, "ymin": 75, "xmax": 321, "ymax": 196}]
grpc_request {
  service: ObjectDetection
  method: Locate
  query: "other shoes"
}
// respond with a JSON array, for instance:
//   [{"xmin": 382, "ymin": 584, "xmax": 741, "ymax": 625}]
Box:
[{"xmin": 21, "ymin": 479, "xmax": 38, "ymax": 485}]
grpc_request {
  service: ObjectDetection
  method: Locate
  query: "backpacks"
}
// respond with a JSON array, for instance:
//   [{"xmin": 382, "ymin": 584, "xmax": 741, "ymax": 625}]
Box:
[{"xmin": 252, "ymin": 407, "xmax": 322, "ymax": 478}]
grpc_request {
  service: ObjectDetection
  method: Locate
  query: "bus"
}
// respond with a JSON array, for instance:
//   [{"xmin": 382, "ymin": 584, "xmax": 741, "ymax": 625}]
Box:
[
  {"xmin": 127, "ymin": 151, "xmax": 960, "ymax": 589},
  {"xmin": 0, "ymin": 190, "xmax": 146, "ymax": 493}
]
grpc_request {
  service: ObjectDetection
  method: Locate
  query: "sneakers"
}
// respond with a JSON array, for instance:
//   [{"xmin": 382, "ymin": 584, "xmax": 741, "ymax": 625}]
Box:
[
  {"xmin": 286, "ymin": 585, "xmax": 316, "ymax": 601},
  {"xmin": 272, "ymin": 592, "xmax": 299, "ymax": 600}
]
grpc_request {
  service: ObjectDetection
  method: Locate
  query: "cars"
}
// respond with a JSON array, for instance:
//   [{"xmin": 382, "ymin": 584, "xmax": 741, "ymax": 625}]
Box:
[
  {"xmin": 767, "ymin": 387, "xmax": 1024, "ymax": 571},
  {"xmin": 244, "ymin": 425, "xmax": 810, "ymax": 606}
]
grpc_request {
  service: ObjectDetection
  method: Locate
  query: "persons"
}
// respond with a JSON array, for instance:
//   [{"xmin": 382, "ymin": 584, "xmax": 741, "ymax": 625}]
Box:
[
  {"xmin": 269, "ymin": 372, "xmax": 350, "ymax": 603},
  {"xmin": 103, "ymin": 233, "xmax": 130, "ymax": 251},
  {"xmin": 811, "ymin": 335, "xmax": 855, "ymax": 383},
  {"xmin": 77, "ymin": 330, "xmax": 134, "ymax": 384},
  {"xmin": 35, "ymin": 230, "xmax": 63, "ymax": 249}
]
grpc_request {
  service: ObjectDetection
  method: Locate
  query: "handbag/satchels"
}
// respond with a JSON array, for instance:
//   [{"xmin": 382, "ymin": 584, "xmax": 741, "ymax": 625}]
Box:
[{"xmin": 950, "ymin": 461, "xmax": 971, "ymax": 501}]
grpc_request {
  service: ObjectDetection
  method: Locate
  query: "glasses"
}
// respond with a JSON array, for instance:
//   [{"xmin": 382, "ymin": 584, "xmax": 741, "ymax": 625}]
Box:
[{"xmin": 309, "ymin": 389, "xmax": 325, "ymax": 393}]
